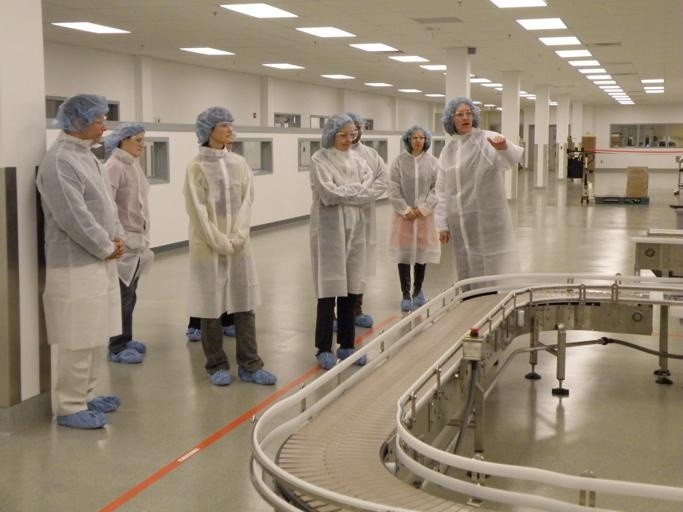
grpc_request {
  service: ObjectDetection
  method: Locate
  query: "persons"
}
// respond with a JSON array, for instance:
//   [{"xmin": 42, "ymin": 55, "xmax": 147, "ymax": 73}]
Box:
[
  {"xmin": 185, "ymin": 313, "xmax": 238, "ymax": 342},
  {"xmin": 34, "ymin": 93, "xmax": 128, "ymax": 430},
  {"xmin": 433, "ymin": 96, "xmax": 525, "ymax": 301},
  {"xmin": 332, "ymin": 112, "xmax": 389, "ymax": 332},
  {"xmin": 388, "ymin": 125, "xmax": 442, "ymax": 312},
  {"xmin": 102, "ymin": 122, "xmax": 155, "ymax": 364},
  {"xmin": 184, "ymin": 106, "xmax": 279, "ymax": 386},
  {"xmin": 308, "ymin": 113, "xmax": 368, "ymax": 370}
]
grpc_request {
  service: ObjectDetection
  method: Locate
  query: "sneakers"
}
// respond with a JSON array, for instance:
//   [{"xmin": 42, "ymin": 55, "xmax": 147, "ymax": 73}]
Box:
[
  {"xmin": 318, "ymin": 313, "xmax": 373, "ymax": 369},
  {"xmin": 186, "ymin": 325, "xmax": 276, "ymax": 385},
  {"xmin": 107, "ymin": 340, "xmax": 145, "ymax": 363},
  {"xmin": 57, "ymin": 397, "xmax": 119, "ymax": 428},
  {"xmin": 401, "ymin": 291, "xmax": 425, "ymax": 311}
]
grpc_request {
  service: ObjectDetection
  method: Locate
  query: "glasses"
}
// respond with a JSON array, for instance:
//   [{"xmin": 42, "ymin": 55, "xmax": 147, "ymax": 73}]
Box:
[
  {"xmin": 453, "ymin": 111, "xmax": 473, "ymax": 120},
  {"xmin": 338, "ymin": 131, "xmax": 359, "ymax": 143},
  {"xmin": 90, "ymin": 119, "xmax": 106, "ymax": 126}
]
